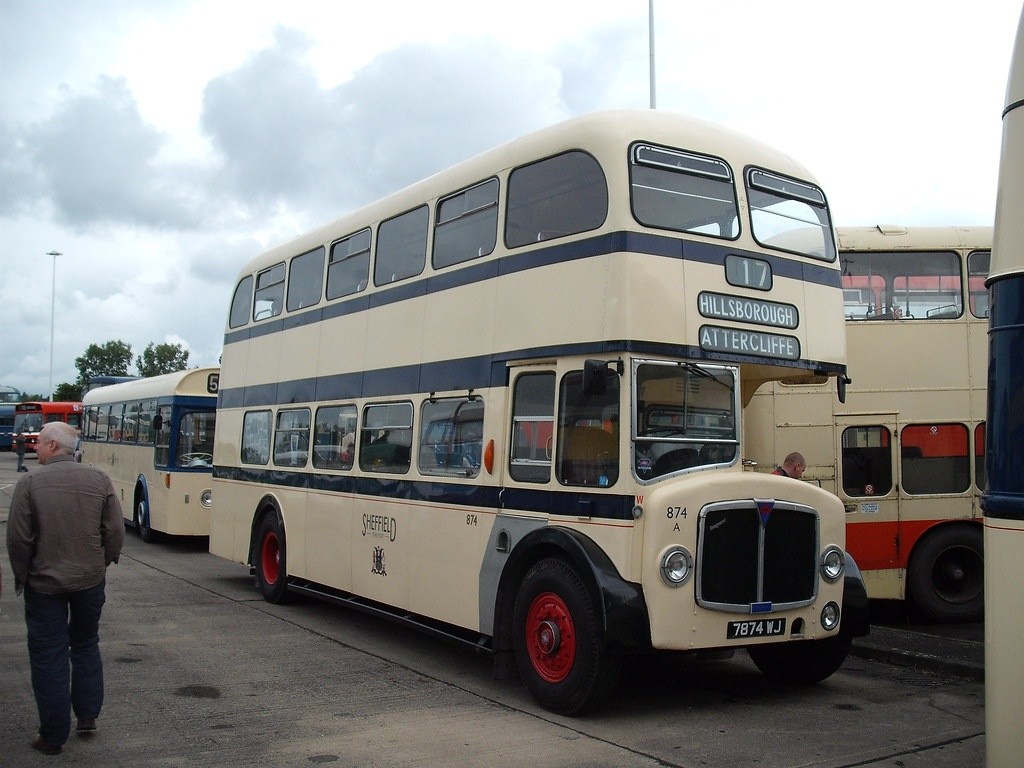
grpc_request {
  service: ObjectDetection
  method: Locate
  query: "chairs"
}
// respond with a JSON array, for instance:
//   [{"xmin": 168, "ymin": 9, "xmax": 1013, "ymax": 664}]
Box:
[
  {"xmin": 299, "ymin": 300, "xmax": 312, "ymax": 308},
  {"xmin": 538, "ymin": 230, "xmax": 571, "ymax": 241},
  {"xmin": 867, "ymin": 307, "xmax": 895, "ymax": 320},
  {"xmin": 927, "ymin": 305, "xmax": 961, "ymax": 318},
  {"xmin": 392, "ymin": 272, "xmax": 412, "ymax": 282},
  {"xmin": 478, "ymin": 245, "xmax": 494, "ymax": 256},
  {"xmin": 357, "ymin": 279, "xmax": 367, "ymax": 291}
]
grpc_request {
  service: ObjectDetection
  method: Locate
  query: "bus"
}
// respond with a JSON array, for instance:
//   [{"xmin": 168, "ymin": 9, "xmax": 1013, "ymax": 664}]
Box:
[
  {"xmin": 0, "ymin": 404, "xmax": 42, "ymax": 450},
  {"xmin": 741, "ymin": 228, "xmax": 997, "ymax": 623},
  {"xmin": 79, "ymin": 367, "xmax": 219, "ymax": 544},
  {"xmin": 12, "ymin": 402, "xmax": 81, "ymax": 452},
  {"xmin": 208, "ymin": 110, "xmax": 872, "ymax": 716}
]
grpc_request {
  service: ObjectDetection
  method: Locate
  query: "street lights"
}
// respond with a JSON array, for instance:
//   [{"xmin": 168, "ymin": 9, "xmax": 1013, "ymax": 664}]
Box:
[{"xmin": 46, "ymin": 250, "xmax": 63, "ymax": 404}]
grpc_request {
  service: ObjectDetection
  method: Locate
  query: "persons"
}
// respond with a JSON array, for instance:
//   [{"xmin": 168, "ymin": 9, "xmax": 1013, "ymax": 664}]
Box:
[
  {"xmin": 6, "ymin": 421, "xmax": 126, "ymax": 754},
  {"xmin": 16, "ymin": 433, "xmax": 27, "ymax": 472},
  {"xmin": 770, "ymin": 452, "xmax": 806, "ymax": 479}
]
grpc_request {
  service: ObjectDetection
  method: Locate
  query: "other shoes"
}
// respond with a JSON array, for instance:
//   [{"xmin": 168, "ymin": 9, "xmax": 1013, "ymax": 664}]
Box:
[
  {"xmin": 76, "ymin": 717, "xmax": 98, "ymax": 738},
  {"xmin": 31, "ymin": 733, "xmax": 63, "ymax": 755},
  {"xmin": 17, "ymin": 469, "xmax": 25, "ymax": 472}
]
